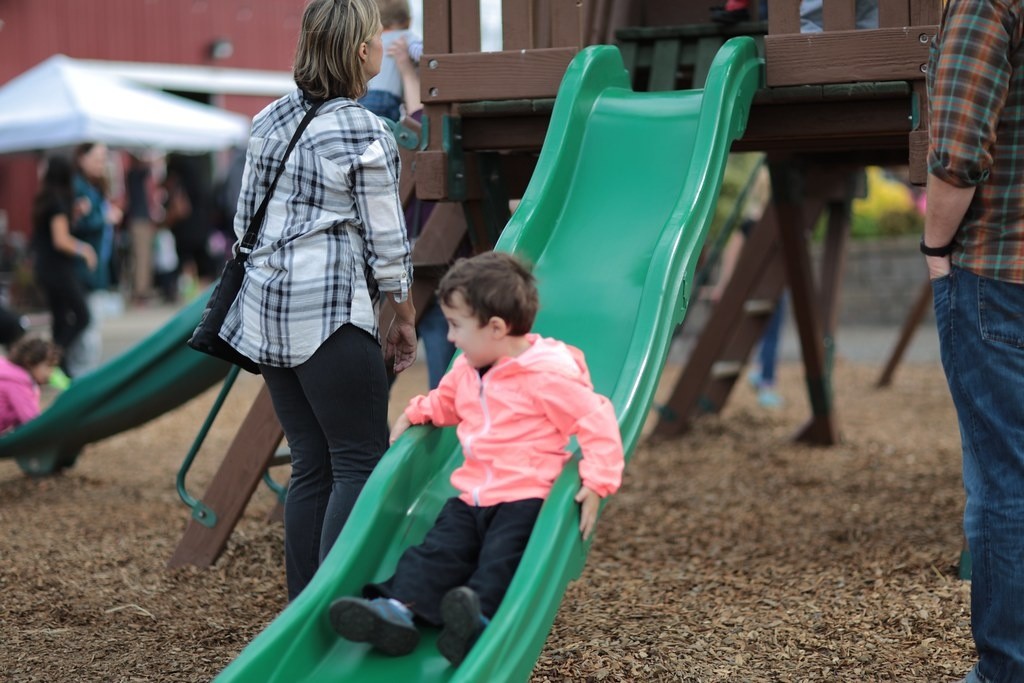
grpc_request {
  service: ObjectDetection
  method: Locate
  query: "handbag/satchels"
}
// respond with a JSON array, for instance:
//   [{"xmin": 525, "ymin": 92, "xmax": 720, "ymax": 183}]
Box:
[{"xmin": 186, "ymin": 245, "xmax": 264, "ymax": 377}]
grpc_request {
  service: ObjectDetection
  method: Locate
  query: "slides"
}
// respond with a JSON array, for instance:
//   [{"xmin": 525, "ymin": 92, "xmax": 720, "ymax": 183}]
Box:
[
  {"xmin": 218, "ymin": 31, "xmax": 762, "ymax": 682},
  {"xmin": 1, "ymin": 291, "xmax": 230, "ymax": 477}
]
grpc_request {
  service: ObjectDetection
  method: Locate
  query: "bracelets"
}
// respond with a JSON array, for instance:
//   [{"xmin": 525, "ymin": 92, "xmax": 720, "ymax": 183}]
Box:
[{"xmin": 921, "ymin": 240, "xmax": 956, "ymax": 257}]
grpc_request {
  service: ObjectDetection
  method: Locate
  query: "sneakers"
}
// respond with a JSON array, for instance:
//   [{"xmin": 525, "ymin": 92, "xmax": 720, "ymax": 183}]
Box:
[
  {"xmin": 437, "ymin": 588, "xmax": 487, "ymax": 665},
  {"xmin": 327, "ymin": 597, "xmax": 420, "ymax": 659}
]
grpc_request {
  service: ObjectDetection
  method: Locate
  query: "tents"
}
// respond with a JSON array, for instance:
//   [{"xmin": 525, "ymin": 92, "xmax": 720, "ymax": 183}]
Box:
[{"xmin": 0, "ymin": 54, "xmax": 250, "ymax": 154}]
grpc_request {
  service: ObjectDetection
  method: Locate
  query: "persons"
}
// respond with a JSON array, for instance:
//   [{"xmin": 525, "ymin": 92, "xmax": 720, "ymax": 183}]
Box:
[
  {"xmin": 708, "ymin": 165, "xmax": 786, "ymax": 407},
  {"xmin": 920, "ymin": 0, "xmax": 1024, "ymax": 683},
  {"xmin": 358, "ymin": 0, "xmax": 462, "ymax": 389},
  {"xmin": 329, "ymin": 253, "xmax": 626, "ymax": 665},
  {"xmin": 220, "ymin": 0, "xmax": 416, "ymax": 605},
  {"xmin": 0, "ymin": 143, "xmax": 246, "ymax": 431}
]
